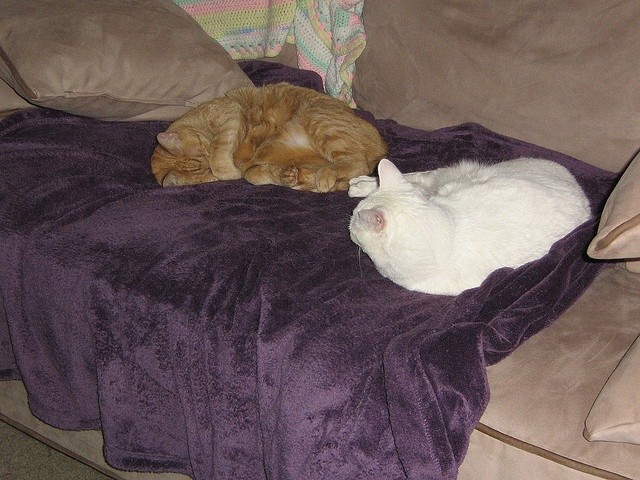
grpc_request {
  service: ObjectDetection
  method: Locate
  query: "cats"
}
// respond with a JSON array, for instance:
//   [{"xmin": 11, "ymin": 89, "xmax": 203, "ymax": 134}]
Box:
[
  {"xmin": 348, "ymin": 158, "xmax": 592, "ymax": 297},
  {"xmin": 150, "ymin": 81, "xmax": 386, "ymax": 193}
]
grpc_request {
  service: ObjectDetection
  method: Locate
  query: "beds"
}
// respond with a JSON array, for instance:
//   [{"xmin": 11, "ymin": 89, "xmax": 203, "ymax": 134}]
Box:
[{"xmin": 0, "ymin": 1, "xmax": 640, "ymax": 480}]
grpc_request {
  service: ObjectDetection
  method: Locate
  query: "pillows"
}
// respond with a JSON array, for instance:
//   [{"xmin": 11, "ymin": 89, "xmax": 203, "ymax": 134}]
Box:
[
  {"xmin": 589, "ymin": 144, "xmax": 637, "ymax": 264},
  {"xmin": 581, "ymin": 331, "xmax": 640, "ymax": 443},
  {"xmin": 0, "ymin": 0, "xmax": 255, "ymax": 120}
]
